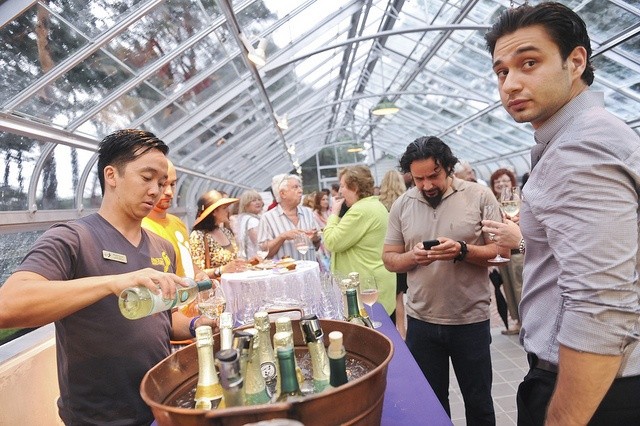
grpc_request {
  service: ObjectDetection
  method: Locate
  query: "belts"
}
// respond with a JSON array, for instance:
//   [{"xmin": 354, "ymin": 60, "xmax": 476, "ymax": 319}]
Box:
[
  {"xmin": 511, "ymin": 249, "xmax": 519, "ymax": 254},
  {"xmin": 527, "ymin": 353, "xmax": 556, "ymax": 373}
]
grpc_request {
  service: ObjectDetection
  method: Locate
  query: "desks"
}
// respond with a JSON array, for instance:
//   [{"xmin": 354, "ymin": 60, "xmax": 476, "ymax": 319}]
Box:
[{"xmin": 151, "ymin": 300, "xmax": 456, "ymax": 425}]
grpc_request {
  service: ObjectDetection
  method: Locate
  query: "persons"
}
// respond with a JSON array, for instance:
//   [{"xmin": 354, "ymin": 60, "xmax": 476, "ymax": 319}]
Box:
[
  {"xmin": 322, "ymin": 189, "xmax": 330, "ymax": 194},
  {"xmin": 381, "ymin": 135, "xmax": 511, "ymax": 426},
  {"xmin": 452, "ymin": 161, "xmax": 475, "ymax": 182},
  {"xmin": 403, "ymin": 172, "xmax": 416, "ymax": 190},
  {"xmin": 373, "ymin": 187, "xmax": 380, "ymax": 195},
  {"xmin": 303, "ymin": 194, "xmax": 315, "ymax": 208},
  {"xmin": 258, "ymin": 174, "xmax": 322, "ymax": 261},
  {"xmin": 219, "ymin": 190, "xmax": 234, "ymax": 237},
  {"xmin": 188, "ymin": 190, "xmax": 247, "ymax": 282},
  {"xmin": 229, "ymin": 201, "xmax": 241, "ymax": 242},
  {"xmin": 484, "ymin": 0, "xmax": 640, "ymax": 426},
  {"xmin": 311, "ymin": 190, "xmax": 317, "ymax": 197},
  {"xmin": 378, "ymin": 170, "xmax": 408, "ymax": 341},
  {"xmin": 313, "ymin": 192, "xmax": 332, "ymax": 230},
  {"xmin": 323, "ymin": 164, "xmax": 396, "ymax": 327},
  {"xmin": 490, "ymin": 168, "xmax": 526, "ymax": 335},
  {"xmin": 0, "ymin": 128, "xmax": 220, "ymax": 426},
  {"xmin": 140, "ymin": 158, "xmax": 211, "ymax": 317},
  {"xmin": 331, "ymin": 184, "xmax": 340, "ymax": 196},
  {"xmin": 238, "ymin": 190, "xmax": 265, "ymax": 259}
]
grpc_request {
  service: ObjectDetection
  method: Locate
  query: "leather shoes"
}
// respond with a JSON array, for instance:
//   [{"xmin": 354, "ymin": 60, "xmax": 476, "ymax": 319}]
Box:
[{"xmin": 501, "ymin": 330, "xmax": 519, "ymax": 335}]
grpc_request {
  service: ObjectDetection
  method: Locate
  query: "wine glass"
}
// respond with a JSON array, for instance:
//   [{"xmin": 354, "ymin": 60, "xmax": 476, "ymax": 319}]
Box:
[
  {"xmin": 294, "ymin": 232, "xmax": 310, "ymax": 267},
  {"xmin": 484, "ymin": 205, "xmax": 511, "ymax": 262},
  {"xmin": 360, "ymin": 289, "xmax": 382, "ymax": 328},
  {"xmin": 198, "ymin": 279, "xmax": 226, "ymax": 334},
  {"xmin": 256, "ymin": 240, "xmax": 269, "ymax": 269},
  {"xmin": 237, "ymin": 249, "xmax": 248, "ymax": 260},
  {"xmin": 501, "ymin": 186, "xmax": 521, "ymax": 221}
]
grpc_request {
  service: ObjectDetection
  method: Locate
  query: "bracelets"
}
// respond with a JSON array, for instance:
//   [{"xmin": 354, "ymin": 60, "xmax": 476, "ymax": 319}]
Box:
[
  {"xmin": 454, "ymin": 241, "xmax": 467, "ymax": 264},
  {"xmin": 215, "ymin": 268, "xmax": 220, "ymax": 277},
  {"xmin": 189, "ymin": 317, "xmax": 200, "ymax": 338},
  {"xmin": 312, "ymin": 237, "xmax": 322, "ymax": 245},
  {"xmin": 518, "ymin": 237, "xmax": 525, "ymax": 255}
]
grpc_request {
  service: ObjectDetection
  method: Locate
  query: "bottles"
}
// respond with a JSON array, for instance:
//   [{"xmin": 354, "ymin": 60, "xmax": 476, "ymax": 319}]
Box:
[
  {"xmin": 216, "ymin": 349, "xmax": 243, "ymax": 407},
  {"xmin": 185, "ymin": 326, "xmax": 226, "ymax": 410},
  {"xmin": 345, "ymin": 289, "xmax": 367, "ymax": 326},
  {"xmin": 253, "ymin": 311, "xmax": 282, "ymax": 400},
  {"xmin": 219, "ymin": 312, "xmax": 232, "ymax": 349},
  {"xmin": 275, "ymin": 316, "xmax": 303, "ymax": 385},
  {"xmin": 348, "ymin": 272, "xmax": 369, "ymax": 328},
  {"xmin": 243, "ymin": 328, "xmax": 272, "ymax": 404},
  {"xmin": 327, "ymin": 331, "xmax": 348, "ymax": 388},
  {"xmin": 274, "ymin": 332, "xmax": 306, "ymax": 401},
  {"xmin": 118, "ymin": 276, "xmax": 212, "ymax": 320},
  {"xmin": 299, "ymin": 315, "xmax": 330, "ymax": 392},
  {"xmin": 233, "ymin": 331, "xmax": 252, "ymax": 376},
  {"xmin": 342, "ymin": 279, "xmax": 352, "ymax": 320}
]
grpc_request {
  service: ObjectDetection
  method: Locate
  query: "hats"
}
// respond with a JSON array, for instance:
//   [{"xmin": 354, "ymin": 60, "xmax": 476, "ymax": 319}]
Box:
[{"xmin": 192, "ymin": 189, "xmax": 239, "ymax": 228}]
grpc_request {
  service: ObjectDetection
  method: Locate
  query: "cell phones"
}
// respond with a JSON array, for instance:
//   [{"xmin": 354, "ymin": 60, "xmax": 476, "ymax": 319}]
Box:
[
  {"xmin": 422, "ymin": 239, "xmax": 439, "ymax": 250},
  {"xmin": 339, "ymin": 203, "xmax": 350, "ymax": 217}
]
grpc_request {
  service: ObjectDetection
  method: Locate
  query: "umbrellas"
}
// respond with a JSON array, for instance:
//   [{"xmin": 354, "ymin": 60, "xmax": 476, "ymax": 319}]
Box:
[{"xmin": 487, "ymin": 268, "xmax": 510, "ymax": 333}]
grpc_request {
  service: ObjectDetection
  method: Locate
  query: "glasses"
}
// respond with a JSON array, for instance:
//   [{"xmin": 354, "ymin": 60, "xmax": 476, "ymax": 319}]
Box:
[{"xmin": 493, "ymin": 179, "xmax": 510, "ymax": 184}]
